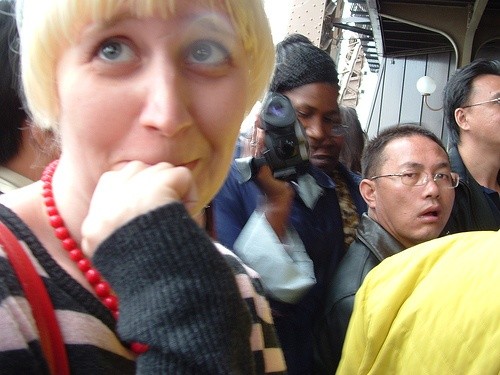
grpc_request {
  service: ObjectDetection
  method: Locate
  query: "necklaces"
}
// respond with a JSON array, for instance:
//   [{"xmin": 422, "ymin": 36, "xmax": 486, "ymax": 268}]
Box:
[{"xmin": 42, "ymin": 158, "xmax": 151, "ymax": 352}]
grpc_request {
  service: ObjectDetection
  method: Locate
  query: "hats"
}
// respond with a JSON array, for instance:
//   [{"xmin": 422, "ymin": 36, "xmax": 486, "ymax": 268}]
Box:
[{"xmin": 268, "ymin": 33, "xmax": 339, "ymax": 91}]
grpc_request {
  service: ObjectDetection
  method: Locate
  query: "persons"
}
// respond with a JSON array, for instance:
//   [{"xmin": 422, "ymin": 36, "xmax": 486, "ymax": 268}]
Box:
[
  {"xmin": 208, "ymin": 33, "xmax": 374, "ymax": 375},
  {"xmin": 311, "ymin": 126, "xmax": 455, "ymax": 375},
  {"xmin": 0, "ymin": 0, "xmax": 62, "ymax": 196},
  {"xmin": 439, "ymin": 59, "xmax": 500, "ymax": 237},
  {"xmin": 0, "ymin": 0, "xmax": 287, "ymax": 375}
]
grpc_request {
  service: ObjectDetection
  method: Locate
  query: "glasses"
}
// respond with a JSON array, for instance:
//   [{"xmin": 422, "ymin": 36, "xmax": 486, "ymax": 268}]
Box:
[{"xmin": 370, "ymin": 169, "xmax": 461, "ymax": 188}]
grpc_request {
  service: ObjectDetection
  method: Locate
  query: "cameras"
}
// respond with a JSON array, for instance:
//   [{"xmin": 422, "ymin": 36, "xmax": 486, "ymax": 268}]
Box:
[{"xmin": 257, "ymin": 92, "xmax": 313, "ymax": 179}]
